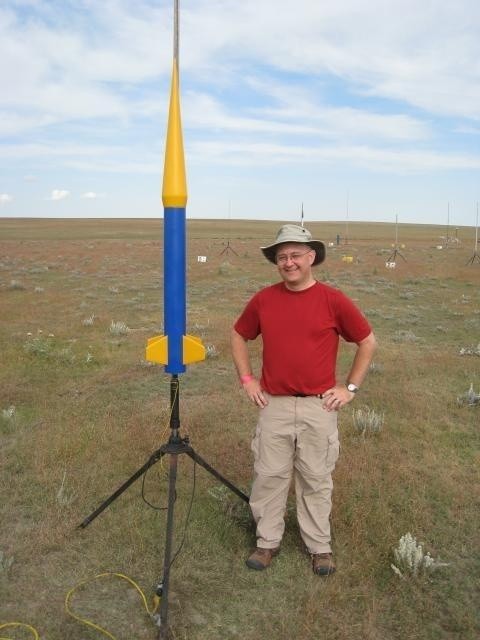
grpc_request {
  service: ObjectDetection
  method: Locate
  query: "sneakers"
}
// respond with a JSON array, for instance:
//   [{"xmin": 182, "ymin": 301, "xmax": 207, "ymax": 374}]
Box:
[
  {"xmin": 312, "ymin": 550, "xmax": 335, "ymax": 576},
  {"xmin": 244, "ymin": 545, "xmax": 281, "ymax": 570}
]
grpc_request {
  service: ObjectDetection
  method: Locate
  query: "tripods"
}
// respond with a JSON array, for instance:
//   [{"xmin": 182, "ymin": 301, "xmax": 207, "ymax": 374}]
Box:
[
  {"xmin": 220, "ymin": 240, "xmax": 239, "ymax": 256},
  {"xmin": 387, "ymin": 249, "xmax": 408, "ymax": 263},
  {"xmin": 76, "ymin": 369, "xmax": 252, "ymax": 640},
  {"xmin": 465, "ymin": 255, "xmax": 480, "ymax": 265}
]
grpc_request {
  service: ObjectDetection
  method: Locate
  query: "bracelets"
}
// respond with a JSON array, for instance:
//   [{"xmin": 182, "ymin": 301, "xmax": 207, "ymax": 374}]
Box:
[{"xmin": 239, "ymin": 373, "xmax": 255, "ymax": 385}]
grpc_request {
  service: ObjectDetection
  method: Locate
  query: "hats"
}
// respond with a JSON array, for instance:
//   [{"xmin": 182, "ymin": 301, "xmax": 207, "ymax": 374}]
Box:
[{"xmin": 259, "ymin": 224, "xmax": 326, "ymax": 268}]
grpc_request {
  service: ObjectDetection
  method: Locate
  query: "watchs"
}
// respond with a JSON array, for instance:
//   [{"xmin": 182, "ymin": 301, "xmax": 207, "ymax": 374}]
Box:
[{"xmin": 344, "ymin": 380, "xmax": 360, "ymax": 394}]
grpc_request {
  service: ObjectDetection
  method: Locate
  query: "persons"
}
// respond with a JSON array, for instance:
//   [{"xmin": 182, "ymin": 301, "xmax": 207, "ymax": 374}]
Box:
[{"xmin": 228, "ymin": 223, "xmax": 379, "ymax": 576}]
